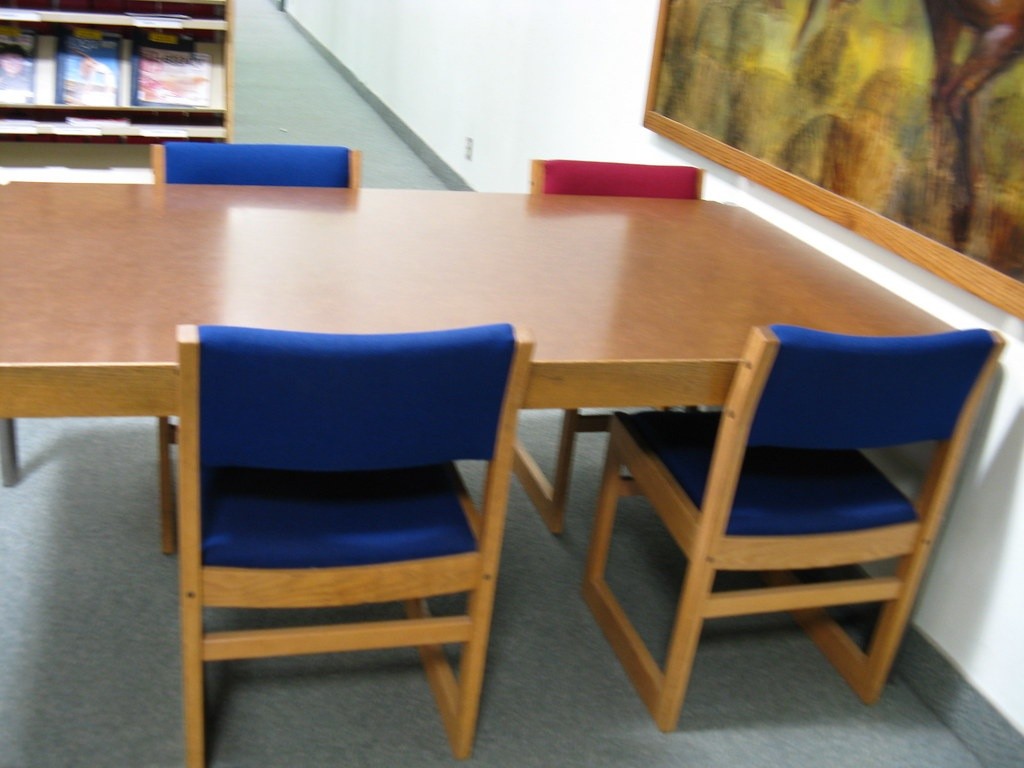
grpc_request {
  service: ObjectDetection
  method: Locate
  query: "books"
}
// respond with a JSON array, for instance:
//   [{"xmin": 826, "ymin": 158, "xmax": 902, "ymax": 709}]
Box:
[{"xmin": 0, "ymin": 26, "xmax": 214, "ymax": 106}]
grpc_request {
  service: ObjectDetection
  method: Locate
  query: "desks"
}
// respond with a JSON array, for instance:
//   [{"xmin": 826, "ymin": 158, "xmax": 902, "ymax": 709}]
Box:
[{"xmin": 0, "ymin": 184, "xmax": 960, "ymax": 489}]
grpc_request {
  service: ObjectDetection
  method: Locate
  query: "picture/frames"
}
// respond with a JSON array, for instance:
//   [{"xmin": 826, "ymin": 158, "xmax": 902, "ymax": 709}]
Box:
[{"xmin": 643, "ymin": 0, "xmax": 1024, "ymax": 319}]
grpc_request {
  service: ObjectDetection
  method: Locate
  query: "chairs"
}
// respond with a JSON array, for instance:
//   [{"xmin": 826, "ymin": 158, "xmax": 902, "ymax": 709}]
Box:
[
  {"xmin": 579, "ymin": 325, "xmax": 1007, "ymax": 731},
  {"xmin": 511, "ymin": 160, "xmax": 707, "ymax": 532},
  {"xmin": 147, "ymin": 141, "xmax": 361, "ymax": 555},
  {"xmin": 172, "ymin": 321, "xmax": 536, "ymax": 767}
]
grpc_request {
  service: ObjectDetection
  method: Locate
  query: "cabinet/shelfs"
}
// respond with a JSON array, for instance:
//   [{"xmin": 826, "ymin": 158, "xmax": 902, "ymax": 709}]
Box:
[{"xmin": 0, "ymin": 0, "xmax": 235, "ymax": 185}]
